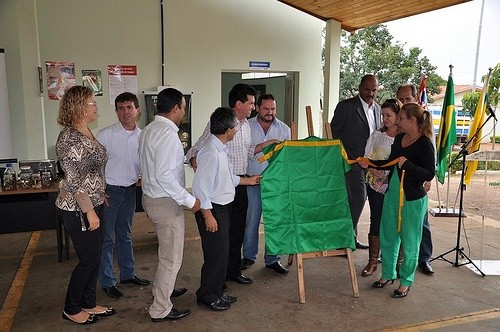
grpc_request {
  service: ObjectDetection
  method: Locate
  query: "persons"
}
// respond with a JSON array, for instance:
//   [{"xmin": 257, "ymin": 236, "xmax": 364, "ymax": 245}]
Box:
[
  {"xmin": 241, "ymin": 94, "xmax": 291, "ymax": 275},
  {"xmin": 54, "ymin": 85, "xmax": 117, "ymax": 325},
  {"xmin": 186, "ymin": 83, "xmax": 280, "ymax": 290},
  {"xmin": 361, "ymin": 98, "xmax": 404, "ymax": 277},
  {"xmin": 371, "ymin": 102, "xmax": 435, "ymax": 298},
  {"xmin": 98, "ymin": 92, "xmax": 149, "ymax": 301},
  {"xmin": 138, "ymin": 87, "xmax": 200, "ymax": 321},
  {"xmin": 398, "ymin": 82, "xmax": 435, "ymax": 275},
  {"xmin": 190, "ymin": 107, "xmax": 262, "ymax": 311},
  {"xmin": 330, "ymin": 74, "xmax": 383, "ymax": 258}
]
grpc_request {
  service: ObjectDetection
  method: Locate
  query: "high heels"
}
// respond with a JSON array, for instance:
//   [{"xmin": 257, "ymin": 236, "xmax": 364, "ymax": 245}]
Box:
[
  {"xmin": 372, "ymin": 278, "xmax": 395, "ymax": 288},
  {"xmin": 393, "ymin": 284, "xmax": 411, "ymax": 298}
]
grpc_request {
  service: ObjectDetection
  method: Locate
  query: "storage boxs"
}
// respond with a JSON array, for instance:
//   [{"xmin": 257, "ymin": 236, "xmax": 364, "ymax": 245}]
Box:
[{"xmin": 19, "ymin": 159, "xmax": 56, "ymax": 179}]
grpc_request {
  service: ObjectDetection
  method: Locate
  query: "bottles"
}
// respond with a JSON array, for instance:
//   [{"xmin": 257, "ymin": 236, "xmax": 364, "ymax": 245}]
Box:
[
  {"xmin": 18, "ymin": 166, "xmax": 33, "ymax": 190},
  {"xmin": 37, "ymin": 159, "xmax": 54, "ymax": 189},
  {"xmin": 3, "ymin": 164, "xmax": 17, "ymax": 191}
]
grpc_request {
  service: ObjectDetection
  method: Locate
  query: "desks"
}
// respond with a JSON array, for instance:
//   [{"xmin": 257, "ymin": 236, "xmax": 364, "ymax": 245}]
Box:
[{"xmin": 0, "ymin": 179, "xmax": 70, "ymax": 262}]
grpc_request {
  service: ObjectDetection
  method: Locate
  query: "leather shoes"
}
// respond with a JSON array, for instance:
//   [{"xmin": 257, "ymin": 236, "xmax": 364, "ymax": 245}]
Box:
[
  {"xmin": 230, "ymin": 273, "xmax": 254, "ymax": 285},
  {"xmin": 170, "ymin": 287, "xmax": 187, "ymax": 298},
  {"xmin": 220, "ymin": 294, "xmax": 237, "ymax": 304},
  {"xmin": 265, "ymin": 261, "xmax": 289, "ymax": 274},
  {"xmin": 151, "ymin": 308, "xmax": 191, "ymax": 322},
  {"xmin": 241, "ymin": 257, "xmax": 255, "ymax": 271},
  {"xmin": 102, "ymin": 286, "xmax": 123, "ymax": 299},
  {"xmin": 121, "ymin": 276, "xmax": 152, "ymax": 286},
  {"xmin": 221, "ymin": 282, "xmax": 229, "ymax": 291},
  {"xmin": 196, "ymin": 297, "xmax": 231, "ymax": 311}
]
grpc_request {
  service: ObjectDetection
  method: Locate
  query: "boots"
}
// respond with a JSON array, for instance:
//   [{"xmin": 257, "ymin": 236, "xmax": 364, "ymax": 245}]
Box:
[
  {"xmin": 395, "ymin": 241, "xmax": 404, "ymax": 278},
  {"xmin": 362, "ymin": 234, "xmax": 380, "ymax": 276}
]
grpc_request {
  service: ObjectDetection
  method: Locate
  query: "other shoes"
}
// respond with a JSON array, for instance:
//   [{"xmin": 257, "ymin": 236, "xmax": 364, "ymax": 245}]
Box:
[
  {"xmin": 85, "ymin": 305, "xmax": 117, "ymax": 317},
  {"xmin": 419, "ymin": 262, "xmax": 434, "ymax": 273},
  {"xmin": 355, "ymin": 239, "xmax": 369, "ymax": 250},
  {"xmin": 62, "ymin": 311, "xmax": 99, "ymax": 325}
]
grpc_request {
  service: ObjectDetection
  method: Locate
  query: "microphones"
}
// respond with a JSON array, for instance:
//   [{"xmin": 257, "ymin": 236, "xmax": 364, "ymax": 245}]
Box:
[{"xmin": 487, "ymin": 104, "xmax": 497, "ymax": 121}]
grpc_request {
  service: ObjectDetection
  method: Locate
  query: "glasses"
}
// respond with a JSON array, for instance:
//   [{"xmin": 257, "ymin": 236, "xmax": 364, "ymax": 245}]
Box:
[
  {"xmin": 88, "ymin": 102, "xmax": 95, "ymax": 106},
  {"xmin": 385, "ymin": 99, "xmax": 400, "ymax": 110}
]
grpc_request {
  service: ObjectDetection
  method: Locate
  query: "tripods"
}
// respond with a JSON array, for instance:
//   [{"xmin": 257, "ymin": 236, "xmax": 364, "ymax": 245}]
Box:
[{"xmin": 427, "ymin": 114, "xmax": 491, "ymax": 277}]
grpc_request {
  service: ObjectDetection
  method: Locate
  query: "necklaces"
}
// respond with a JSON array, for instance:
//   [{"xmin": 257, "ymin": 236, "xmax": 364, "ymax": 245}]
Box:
[{"xmin": 405, "ymin": 133, "xmax": 421, "ymax": 144}]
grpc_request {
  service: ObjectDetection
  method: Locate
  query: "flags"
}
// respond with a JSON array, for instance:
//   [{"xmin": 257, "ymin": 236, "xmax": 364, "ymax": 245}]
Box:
[
  {"xmin": 417, "ymin": 74, "xmax": 428, "ymax": 111},
  {"xmin": 435, "ymin": 73, "xmax": 455, "ymax": 185},
  {"xmin": 465, "ymin": 75, "xmax": 492, "ymax": 186}
]
